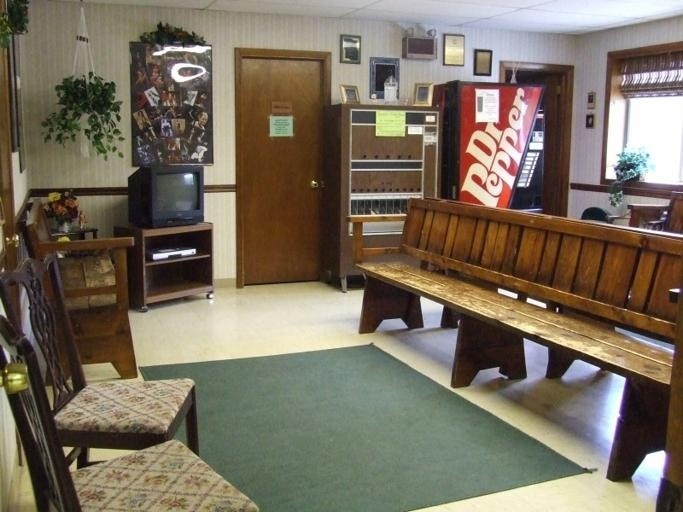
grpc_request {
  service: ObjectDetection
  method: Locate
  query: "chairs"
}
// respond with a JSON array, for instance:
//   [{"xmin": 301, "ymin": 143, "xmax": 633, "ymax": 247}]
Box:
[
  {"xmin": 1, "ymin": 254, "xmax": 199, "ymax": 468},
  {"xmin": 1, "ymin": 316, "xmax": 259, "ymax": 512},
  {"xmin": 628, "ymin": 191, "xmax": 683, "ymax": 234}
]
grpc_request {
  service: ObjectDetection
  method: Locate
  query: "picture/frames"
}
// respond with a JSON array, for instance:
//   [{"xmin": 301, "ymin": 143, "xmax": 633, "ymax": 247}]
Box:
[
  {"xmin": 340, "ymin": 35, "xmax": 361, "ymax": 64},
  {"xmin": 370, "ymin": 57, "xmax": 400, "ymax": 100},
  {"xmin": 413, "ymin": 82, "xmax": 434, "ymax": 106},
  {"xmin": 473, "ymin": 49, "xmax": 492, "ymax": 76},
  {"xmin": 340, "ymin": 85, "xmax": 359, "ymax": 104},
  {"xmin": 443, "ymin": 34, "xmax": 465, "ymax": 66}
]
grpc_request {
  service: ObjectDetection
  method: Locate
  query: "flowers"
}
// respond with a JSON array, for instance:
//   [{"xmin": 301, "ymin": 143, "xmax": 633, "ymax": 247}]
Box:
[
  {"xmin": 608, "ymin": 151, "xmax": 650, "ymax": 207},
  {"xmin": 43, "ymin": 189, "xmax": 81, "ymax": 217}
]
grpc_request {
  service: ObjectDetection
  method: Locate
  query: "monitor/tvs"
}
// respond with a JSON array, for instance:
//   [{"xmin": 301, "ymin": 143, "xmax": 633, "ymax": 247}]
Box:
[{"xmin": 128, "ymin": 166, "xmax": 204, "ymax": 228}]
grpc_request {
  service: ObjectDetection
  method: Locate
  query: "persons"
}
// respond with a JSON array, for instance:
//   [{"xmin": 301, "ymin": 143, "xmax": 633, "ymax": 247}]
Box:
[{"xmin": 192, "ymin": 112, "xmax": 208, "ymax": 129}]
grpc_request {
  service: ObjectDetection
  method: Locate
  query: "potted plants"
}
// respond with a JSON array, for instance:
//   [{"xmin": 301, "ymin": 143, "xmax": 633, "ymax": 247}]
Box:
[
  {"xmin": 140, "ymin": 22, "xmax": 207, "ymax": 48},
  {"xmin": 41, "ymin": 8, "xmax": 126, "ymax": 161},
  {"xmin": 0, "ymin": 0, "xmax": 29, "ymax": 49}
]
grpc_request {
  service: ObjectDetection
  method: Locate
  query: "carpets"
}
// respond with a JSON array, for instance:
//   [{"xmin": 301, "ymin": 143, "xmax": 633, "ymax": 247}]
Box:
[{"xmin": 139, "ymin": 343, "xmax": 592, "ymax": 512}]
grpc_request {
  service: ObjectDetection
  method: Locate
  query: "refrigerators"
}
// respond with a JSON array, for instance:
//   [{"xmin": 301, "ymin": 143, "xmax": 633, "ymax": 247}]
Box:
[{"xmin": 432, "ymin": 81, "xmax": 544, "ymax": 214}]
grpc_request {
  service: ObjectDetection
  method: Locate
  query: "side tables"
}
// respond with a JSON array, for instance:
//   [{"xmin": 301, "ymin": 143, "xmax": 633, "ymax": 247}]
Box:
[{"xmin": 51, "ymin": 227, "xmax": 98, "ymax": 239}]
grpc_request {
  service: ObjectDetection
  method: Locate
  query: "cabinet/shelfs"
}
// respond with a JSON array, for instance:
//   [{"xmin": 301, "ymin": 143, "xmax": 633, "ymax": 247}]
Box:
[{"xmin": 114, "ymin": 222, "xmax": 215, "ymax": 313}]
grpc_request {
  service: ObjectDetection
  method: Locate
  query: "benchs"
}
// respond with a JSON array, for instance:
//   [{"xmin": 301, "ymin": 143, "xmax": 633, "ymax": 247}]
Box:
[
  {"xmin": 347, "ymin": 199, "xmax": 683, "ymax": 512},
  {"xmin": 25, "ymin": 199, "xmax": 137, "ymax": 380}
]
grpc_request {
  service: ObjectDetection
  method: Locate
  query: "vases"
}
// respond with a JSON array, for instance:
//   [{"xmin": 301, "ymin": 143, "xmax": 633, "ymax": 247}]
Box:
[
  {"xmin": 54, "ymin": 219, "xmax": 73, "ymax": 234},
  {"xmin": 616, "ymin": 172, "xmax": 646, "ymax": 186}
]
